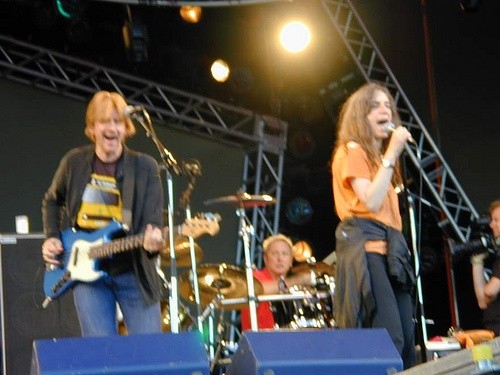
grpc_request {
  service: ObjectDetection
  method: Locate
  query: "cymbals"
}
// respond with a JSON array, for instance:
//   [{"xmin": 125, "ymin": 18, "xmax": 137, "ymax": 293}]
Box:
[
  {"xmin": 160, "ymin": 234, "xmax": 204, "ymax": 268},
  {"xmin": 291, "ymin": 262, "xmax": 336, "ymax": 276},
  {"xmin": 178, "ymin": 261, "xmax": 266, "ymax": 312},
  {"xmin": 202, "ymin": 193, "xmax": 277, "ymax": 207}
]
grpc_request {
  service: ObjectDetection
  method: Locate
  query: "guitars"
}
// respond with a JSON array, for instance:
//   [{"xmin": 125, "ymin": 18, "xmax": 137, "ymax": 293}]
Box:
[{"xmin": 43, "ymin": 210, "xmax": 221, "ymax": 300}]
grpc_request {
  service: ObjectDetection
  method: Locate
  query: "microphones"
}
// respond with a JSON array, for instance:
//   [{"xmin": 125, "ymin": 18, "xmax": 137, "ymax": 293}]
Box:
[
  {"xmin": 125, "ymin": 105, "xmax": 143, "ymax": 117},
  {"xmin": 383, "ymin": 121, "xmax": 413, "ymax": 143}
]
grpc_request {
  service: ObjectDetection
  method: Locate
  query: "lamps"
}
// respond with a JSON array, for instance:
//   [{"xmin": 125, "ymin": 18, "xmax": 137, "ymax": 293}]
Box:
[
  {"xmin": 195, "ymin": 56, "xmax": 231, "ymax": 89},
  {"xmin": 282, "ymin": 168, "xmax": 321, "ymax": 236}
]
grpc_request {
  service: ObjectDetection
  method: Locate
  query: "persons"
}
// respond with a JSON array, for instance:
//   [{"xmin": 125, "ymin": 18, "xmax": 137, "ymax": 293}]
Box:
[
  {"xmin": 41, "ymin": 91, "xmax": 166, "ymax": 340},
  {"xmin": 468, "ymin": 202, "xmax": 500, "ymax": 309},
  {"xmin": 332, "ymin": 84, "xmax": 419, "ymax": 368},
  {"xmin": 241, "ymin": 234, "xmax": 312, "ymax": 336}
]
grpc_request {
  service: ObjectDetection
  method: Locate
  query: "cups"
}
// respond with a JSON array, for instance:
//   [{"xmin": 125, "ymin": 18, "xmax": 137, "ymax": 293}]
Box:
[{"xmin": 15, "ymin": 215, "xmax": 30, "ymax": 234}]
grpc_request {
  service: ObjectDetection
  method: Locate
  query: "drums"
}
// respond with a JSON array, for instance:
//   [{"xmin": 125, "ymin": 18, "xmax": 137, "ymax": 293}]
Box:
[{"xmin": 268, "ymin": 285, "xmax": 327, "ymax": 331}]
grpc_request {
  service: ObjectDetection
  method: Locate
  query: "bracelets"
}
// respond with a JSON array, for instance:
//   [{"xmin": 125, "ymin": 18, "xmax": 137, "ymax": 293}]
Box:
[{"xmin": 471, "ymin": 256, "xmax": 482, "ymax": 265}]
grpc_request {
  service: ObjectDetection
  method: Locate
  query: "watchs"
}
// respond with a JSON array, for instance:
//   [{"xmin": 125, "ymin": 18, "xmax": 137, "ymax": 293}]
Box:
[{"xmin": 382, "ymin": 159, "xmax": 395, "ymax": 168}]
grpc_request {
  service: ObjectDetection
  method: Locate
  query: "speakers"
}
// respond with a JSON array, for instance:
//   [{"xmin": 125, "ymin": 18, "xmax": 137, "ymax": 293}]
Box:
[
  {"xmin": 0, "ymin": 235, "xmax": 82, "ymax": 375},
  {"xmin": 228, "ymin": 328, "xmax": 403, "ymax": 375},
  {"xmin": 29, "ymin": 330, "xmax": 211, "ymax": 375}
]
